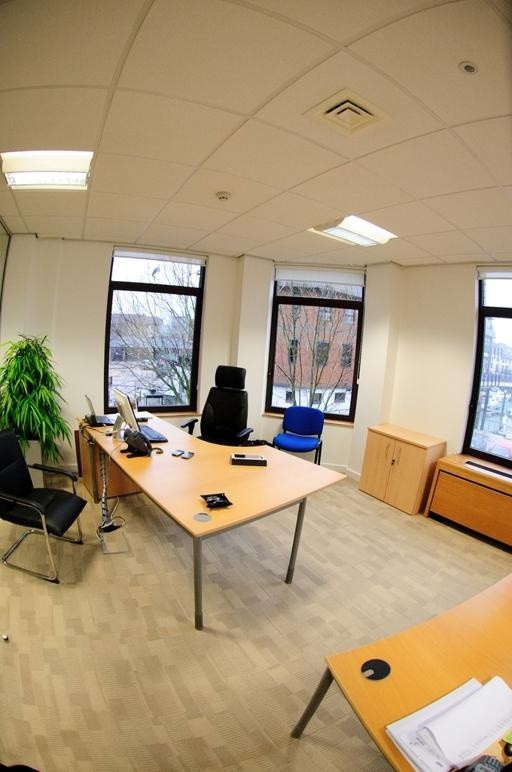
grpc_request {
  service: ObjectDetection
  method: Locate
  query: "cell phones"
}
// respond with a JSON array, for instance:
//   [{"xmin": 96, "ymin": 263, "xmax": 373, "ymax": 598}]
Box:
[
  {"xmin": 182, "ymin": 451, "xmax": 195, "ymax": 459},
  {"xmin": 172, "ymin": 449, "xmax": 183, "ymax": 456}
]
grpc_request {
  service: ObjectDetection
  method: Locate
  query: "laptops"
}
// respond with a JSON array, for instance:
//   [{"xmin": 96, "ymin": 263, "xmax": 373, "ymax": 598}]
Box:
[{"xmin": 85, "ymin": 394, "xmax": 115, "ymax": 427}]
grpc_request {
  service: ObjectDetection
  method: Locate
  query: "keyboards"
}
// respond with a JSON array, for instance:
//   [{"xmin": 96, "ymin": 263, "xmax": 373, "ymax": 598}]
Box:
[{"xmin": 141, "ymin": 425, "xmax": 168, "ymax": 442}]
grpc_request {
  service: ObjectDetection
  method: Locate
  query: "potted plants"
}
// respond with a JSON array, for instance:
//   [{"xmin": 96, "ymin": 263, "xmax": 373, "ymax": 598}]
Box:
[{"xmin": 0, "ymin": 329, "xmax": 73, "ymax": 488}]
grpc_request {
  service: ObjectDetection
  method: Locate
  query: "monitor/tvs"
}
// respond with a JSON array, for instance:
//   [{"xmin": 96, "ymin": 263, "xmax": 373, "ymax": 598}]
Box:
[{"xmin": 112, "ymin": 387, "xmax": 141, "ymax": 442}]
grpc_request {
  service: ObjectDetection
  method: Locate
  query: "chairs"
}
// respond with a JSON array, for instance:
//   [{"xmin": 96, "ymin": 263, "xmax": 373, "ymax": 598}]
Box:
[
  {"xmin": 180, "ymin": 365, "xmax": 254, "ymax": 446},
  {"xmin": 273, "ymin": 406, "xmax": 324, "ymax": 465},
  {"xmin": 0, "ymin": 429, "xmax": 87, "ymax": 581}
]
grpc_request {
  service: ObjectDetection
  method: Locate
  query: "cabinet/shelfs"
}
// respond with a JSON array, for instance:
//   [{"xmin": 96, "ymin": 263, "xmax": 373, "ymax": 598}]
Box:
[
  {"xmin": 358, "ymin": 424, "xmax": 446, "ymax": 516},
  {"xmin": 424, "ymin": 453, "xmax": 512, "ymax": 547}
]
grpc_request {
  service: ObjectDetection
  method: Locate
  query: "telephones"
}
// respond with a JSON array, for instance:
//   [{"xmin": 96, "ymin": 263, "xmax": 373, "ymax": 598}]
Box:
[{"xmin": 120, "ymin": 428, "xmax": 163, "ymax": 458}]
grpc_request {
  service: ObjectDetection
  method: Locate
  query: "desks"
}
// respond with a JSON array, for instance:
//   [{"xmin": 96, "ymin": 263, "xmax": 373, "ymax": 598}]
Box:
[
  {"xmin": 289, "ymin": 571, "xmax": 512, "ymax": 772},
  {"xmin": 77, "ymin": 412, "xmax": 348, "ymax": 630}
]
